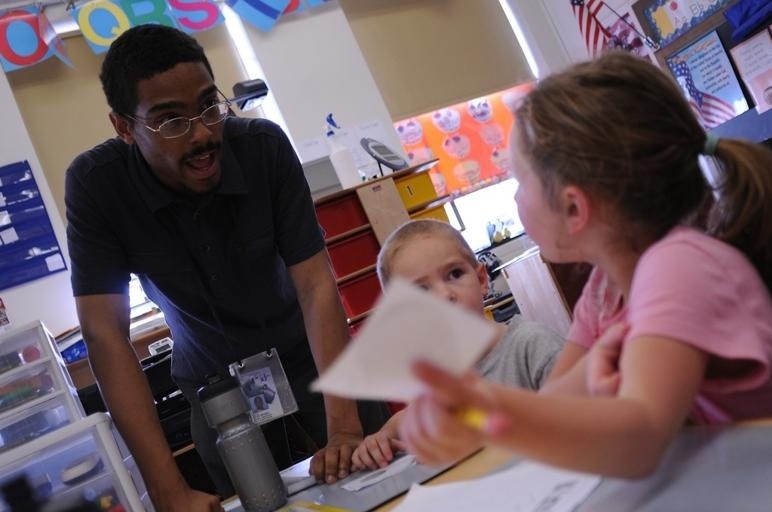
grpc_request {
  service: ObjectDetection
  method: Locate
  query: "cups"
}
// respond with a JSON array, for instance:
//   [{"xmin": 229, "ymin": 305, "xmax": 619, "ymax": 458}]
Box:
[{"xmin": 196, "ymin": 374, "xmax": 289, "ymax": 512}]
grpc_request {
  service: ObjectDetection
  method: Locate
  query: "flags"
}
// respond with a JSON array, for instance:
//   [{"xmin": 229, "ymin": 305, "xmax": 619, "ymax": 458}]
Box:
[
  {"xmin": 570, "ymin": 0, "xmax": 611, "ymax": 62},
  {"xmin": 667, "ymin": 53, "xmax": 736, "ymax": 133}
]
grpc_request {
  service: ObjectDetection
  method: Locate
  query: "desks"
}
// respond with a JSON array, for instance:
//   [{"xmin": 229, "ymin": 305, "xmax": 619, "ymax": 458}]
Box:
[
  {"xmin": 492, "ymin": 242, "xmax": 573, "ymax": 338},
  {"xmin": 192, "ymin": 416, "xmax": 771, "ymax": 512}
]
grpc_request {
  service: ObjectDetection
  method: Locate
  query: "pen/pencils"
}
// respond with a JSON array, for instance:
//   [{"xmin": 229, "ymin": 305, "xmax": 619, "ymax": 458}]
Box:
[{"xmin": 448, "ymin": 409, "xmax": 507, "ymax": 438}]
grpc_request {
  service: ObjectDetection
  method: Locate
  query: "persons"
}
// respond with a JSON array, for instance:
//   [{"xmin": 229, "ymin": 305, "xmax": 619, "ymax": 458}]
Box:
[
  {"xmin": 251, "ymin": 395, "xmax": 266, "ymax": 413},
  {"xmin": 396, "ymin": 48, "xmax": 770, "ymax": 482},
  {"xmin": 344, "ymin": 217, "xmax": 568, "ymax": 473},
  {"xmin": 64, "ymin": 22, "xmax": 366, "ymax": 512}
]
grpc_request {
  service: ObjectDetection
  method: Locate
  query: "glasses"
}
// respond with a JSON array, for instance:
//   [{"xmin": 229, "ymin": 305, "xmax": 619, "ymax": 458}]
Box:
[{"xmin": 123, "ymin": 88, "xmax": 232, "ymax": 140}]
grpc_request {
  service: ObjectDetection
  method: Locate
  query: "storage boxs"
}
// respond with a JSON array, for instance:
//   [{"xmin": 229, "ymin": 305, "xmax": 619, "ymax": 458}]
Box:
[{"xmin": 1, "ymin": 319, "xmax": 155, "ymax": 512}]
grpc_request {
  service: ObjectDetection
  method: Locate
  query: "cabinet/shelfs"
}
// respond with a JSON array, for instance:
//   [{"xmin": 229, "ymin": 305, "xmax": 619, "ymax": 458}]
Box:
[
  {"xmin": 623, "ymin": 1, "xmax": 772, "ymax": 198},
  {"xmin": 66, "ymin": 323, "xmax": 195, "ymax": 457},
  {"xmin": 298, "ymin": 154, "xmax": 462, "ymax": 336}
]
